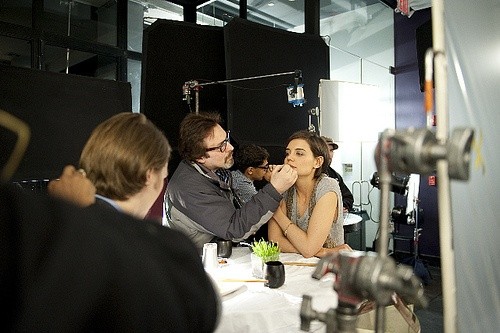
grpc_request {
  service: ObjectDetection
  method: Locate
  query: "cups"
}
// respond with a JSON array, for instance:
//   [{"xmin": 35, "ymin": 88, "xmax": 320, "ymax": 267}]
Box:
[
  {"xmin": 200, "ymin": 242, "xmax": 217, "ymax": 267},
  {"xmin": 216, "ymin": 240, "xmax": 232, "ymax": 258},
  {"xmin": 262, "ymin": 261, "xmax": 285, "ymax": 289}
]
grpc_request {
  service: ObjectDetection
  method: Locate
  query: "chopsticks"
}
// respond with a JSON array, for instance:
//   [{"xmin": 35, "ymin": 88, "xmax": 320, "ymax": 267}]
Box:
[
  {"xmin": 214, "ymin": 279, "xmax": 269, "ymax": 282},
  {"xmin": 281, "ymin": 262, "xmax": 318, "ymax": 267}
]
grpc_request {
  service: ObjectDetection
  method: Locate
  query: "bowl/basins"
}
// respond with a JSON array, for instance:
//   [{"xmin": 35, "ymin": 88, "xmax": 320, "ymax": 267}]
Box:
[{"xmin": 250, "ymin": 242, "xmax": 277, "ymax": 252}]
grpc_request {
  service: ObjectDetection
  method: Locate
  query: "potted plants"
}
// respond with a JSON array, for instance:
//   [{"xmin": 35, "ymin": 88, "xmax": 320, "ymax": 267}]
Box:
[{"xmin": 250, "ymin": 236, "xmax": 280, "ymax": 280}]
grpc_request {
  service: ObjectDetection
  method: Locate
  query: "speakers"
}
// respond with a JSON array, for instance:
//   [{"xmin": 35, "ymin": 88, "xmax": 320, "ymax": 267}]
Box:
[{"xmin": 416, "ymin": 19, "xmax": 435, "ymax": 92}]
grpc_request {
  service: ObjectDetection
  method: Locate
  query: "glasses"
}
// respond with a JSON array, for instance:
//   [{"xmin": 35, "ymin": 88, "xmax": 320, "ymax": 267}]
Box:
[
  {"xmin": 205, "ymin": 129, "xmax": 230, "ymax": 153},
  {"xmin": 256, "ymin": 162, "xmax": 269, "ymax": 170}
]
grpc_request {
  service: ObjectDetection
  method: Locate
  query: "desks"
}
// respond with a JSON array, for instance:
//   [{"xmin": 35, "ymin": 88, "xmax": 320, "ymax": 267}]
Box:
[{"xmin": 201, "ymin": 246, "xmax": 339, "ymax": 333}]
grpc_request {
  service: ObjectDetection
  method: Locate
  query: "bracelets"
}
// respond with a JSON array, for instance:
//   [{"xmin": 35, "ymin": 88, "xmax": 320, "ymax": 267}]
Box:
[{"xmin": 283, "ymin": 222, "xmax": 296, "ymax": 236}]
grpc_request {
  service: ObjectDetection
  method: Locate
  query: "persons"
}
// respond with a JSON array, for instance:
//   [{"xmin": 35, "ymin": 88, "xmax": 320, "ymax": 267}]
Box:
[
  {"xmin": 0, "ymin": 111, "xmax": 221, "ymax": 333},
  {"xmin": 226, "ymin": 130, "xmax": 354, "ymax": 259},
  {"xmin": 162, "ymin": 112, "xmax": 299, "ymax": 262}
]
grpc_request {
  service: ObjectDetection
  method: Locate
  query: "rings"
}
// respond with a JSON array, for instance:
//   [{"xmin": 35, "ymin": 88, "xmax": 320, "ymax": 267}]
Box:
[{"xmin": 77, "ymin": 168, "xmax": 87, "ymax": 178}]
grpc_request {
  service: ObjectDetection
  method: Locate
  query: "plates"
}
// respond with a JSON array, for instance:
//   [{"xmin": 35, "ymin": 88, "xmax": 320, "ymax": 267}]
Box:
[{"xmin": 211, "ymin": 280, "xmax": 243, "ymax": 297}]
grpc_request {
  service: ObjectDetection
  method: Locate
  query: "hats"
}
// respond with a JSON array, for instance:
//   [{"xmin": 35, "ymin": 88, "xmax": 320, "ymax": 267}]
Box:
[{"xmin": 321, "ymin": 136, "xmax": 338, "ymax": 150}]
